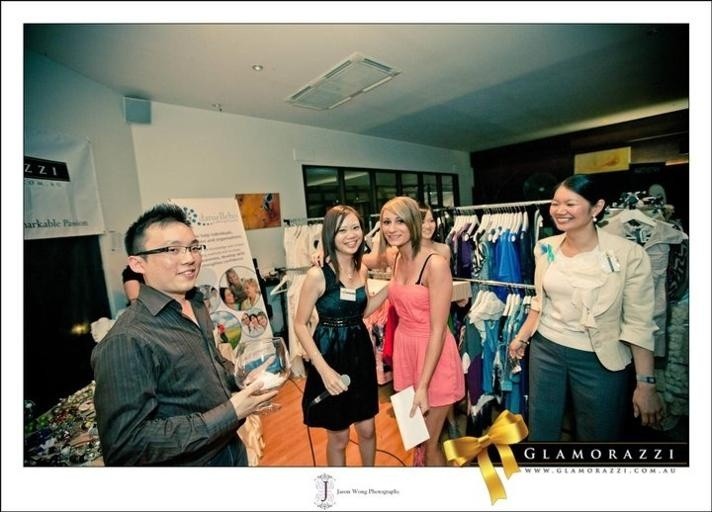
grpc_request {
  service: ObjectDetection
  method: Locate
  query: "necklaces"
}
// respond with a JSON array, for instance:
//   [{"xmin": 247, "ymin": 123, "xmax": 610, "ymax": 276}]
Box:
[{"xmin": 341, "ymin": 265, "xmax": 354, "ymax": 279}]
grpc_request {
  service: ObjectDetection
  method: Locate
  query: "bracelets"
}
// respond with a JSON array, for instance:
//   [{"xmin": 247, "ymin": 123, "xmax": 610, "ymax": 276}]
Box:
[
  {"xmin": 635, "ymin": 373, "xmax": 657, "ymax": 385},
  {"xmin": 515, "ymin": 335, "xmax": 530, "ymax": 346}
]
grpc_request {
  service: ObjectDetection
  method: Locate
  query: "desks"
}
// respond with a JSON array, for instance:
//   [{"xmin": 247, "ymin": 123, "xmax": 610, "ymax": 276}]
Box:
[{"xmin": 24, "ymin": 382, "xmax": 104, "ymax": 467}]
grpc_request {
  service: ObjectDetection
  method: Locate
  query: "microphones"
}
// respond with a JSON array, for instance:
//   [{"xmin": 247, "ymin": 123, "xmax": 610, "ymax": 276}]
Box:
[{"xmin": 310, "ymin": 374, "xmax": 350, "ymax": 405}]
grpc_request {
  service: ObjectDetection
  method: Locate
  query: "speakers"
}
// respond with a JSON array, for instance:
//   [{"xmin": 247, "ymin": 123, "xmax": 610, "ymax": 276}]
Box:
[{"xmin": 122, "ymin": 96, "xmax": 153, "ymax": 125}]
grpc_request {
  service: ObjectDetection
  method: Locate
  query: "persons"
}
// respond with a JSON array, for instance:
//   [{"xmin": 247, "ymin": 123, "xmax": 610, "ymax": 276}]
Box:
[
  {"xmin": 203, "ymin": 268, "xmax": 267, "ymax": 349},
  {"xmin": 293, "ymin": 203, "xmax": 389, "ymax": 467},
  {"xmin": 118, "ymin": 261, "xmax": 142, "ymax": 306},
  {"xmin": 419, "ymin": 202, "xmax": 451, "ymax": 267},
  {"xmin": 509, "ymin": 174, "xmax": 663, "ymax": 444},
  {"xmin": 89, "ymin": 205, "xmax": 292, "ymax": 467},
  {"xmin": 310, "ymin": 196, "xmax": 466, "ymax": 467},
  {"xmin": 262, "ymin": 193, "xmax": 273, "ymax": 212}
]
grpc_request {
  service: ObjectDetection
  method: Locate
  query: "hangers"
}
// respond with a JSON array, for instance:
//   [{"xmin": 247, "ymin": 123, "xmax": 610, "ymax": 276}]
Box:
[
  {"xmin": 451, "ymin": 198, "xmax": 544, "ymax": 243},
  {"xmin": 468, "ymin": 283, "xmax": 536, "ymax": 324},
  {"xmin": 363, "ymin": 220, "xmax": 383, "ymax": 240},
  {"xmin": 595, "ymin": 194, "xmax": 688, "ymax": 240}
]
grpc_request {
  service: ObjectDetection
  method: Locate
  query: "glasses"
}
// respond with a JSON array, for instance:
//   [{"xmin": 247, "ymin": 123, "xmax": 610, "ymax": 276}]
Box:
[{"xmin": 134, "ymin": 244, "xmax": 207, "ymax": 257}]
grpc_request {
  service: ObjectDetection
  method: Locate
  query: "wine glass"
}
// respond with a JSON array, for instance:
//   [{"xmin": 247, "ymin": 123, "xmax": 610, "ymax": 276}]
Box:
[{"xmin": 233, "ymin": 336, "xmax": 293, "ymax": 416}]
